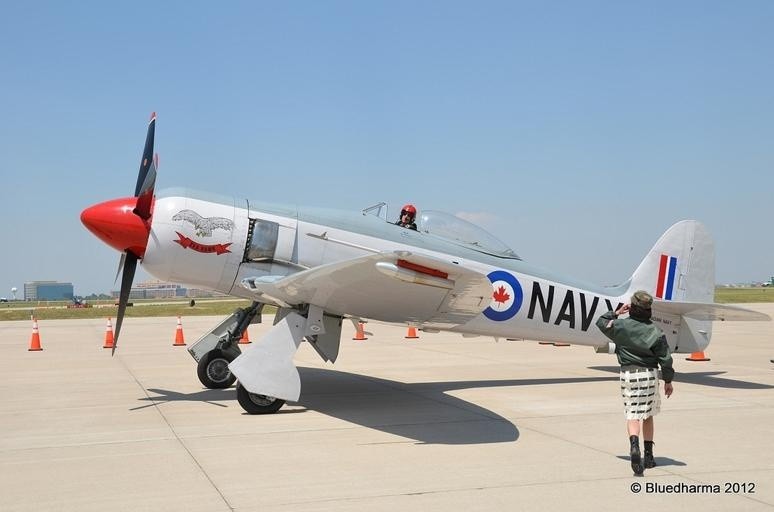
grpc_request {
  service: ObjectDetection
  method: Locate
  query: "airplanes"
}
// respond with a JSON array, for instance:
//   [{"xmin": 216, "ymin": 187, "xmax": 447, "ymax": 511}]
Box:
[{"xmin": 80, "ymin": 110, "xmax": 774, "ymax": 415}]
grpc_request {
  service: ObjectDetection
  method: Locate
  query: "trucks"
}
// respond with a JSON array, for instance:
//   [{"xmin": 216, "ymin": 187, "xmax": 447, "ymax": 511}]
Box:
[{"xmin": 762, "ymin": 279, "xmax": 774, "ymax": 287}]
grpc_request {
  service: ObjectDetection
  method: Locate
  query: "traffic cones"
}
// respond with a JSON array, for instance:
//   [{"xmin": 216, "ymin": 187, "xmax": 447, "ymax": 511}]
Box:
[
  {"xmin": 171, "ymin": 316, "xmax": 187, "ymax": 347},
  {"xmin": 27, "ymin": 319, "xmax": 44, "ymax": 351},
  {"xmin": 101, "ymin": 317, "xmax": 117, "ymax": 348},
  {"xmin": 685, "ymin": 351, "xmax": 712, "ymax": 361},
  {"xmin": 239, "ymin": 327, "xmax": 250, "ymax": 344},
  {"xmin": 352, "ymin": 321, "xmax": 368, "ymax": 340},
  {"xmin": 404, "ymin": 326, "xmax": 419, "ymax": 338}
]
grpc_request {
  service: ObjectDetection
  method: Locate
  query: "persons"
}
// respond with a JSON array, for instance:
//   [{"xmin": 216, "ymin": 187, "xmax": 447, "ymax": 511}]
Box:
[
  {"xmin": 595, "ymin": 289, "xmax": 676, "ymax": 476},
  {"xmin": 395, "ymin": 204, "xmax": 418, "ymax": 231}
]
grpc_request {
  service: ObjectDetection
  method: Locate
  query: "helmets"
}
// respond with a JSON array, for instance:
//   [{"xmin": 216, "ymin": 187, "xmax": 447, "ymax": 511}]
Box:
[{"xmin": 399, "ymin": 203, "xmax": 417, "ymax": 222}]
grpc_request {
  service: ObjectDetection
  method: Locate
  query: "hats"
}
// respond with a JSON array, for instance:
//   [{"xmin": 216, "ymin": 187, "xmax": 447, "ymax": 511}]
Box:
[{"xmin": 630, "ymin": 290, "xmax": 653, "ymax": 309}]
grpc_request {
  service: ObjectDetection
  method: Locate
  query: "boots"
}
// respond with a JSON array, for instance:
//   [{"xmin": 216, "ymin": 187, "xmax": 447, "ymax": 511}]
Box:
[
  {"xmin": 643, "ymin": 440, "xmax": 657, "ymax": 468},
  {"xmin": 629, "ymin": 435, "xmax": 643, "ymax": 474}
]
